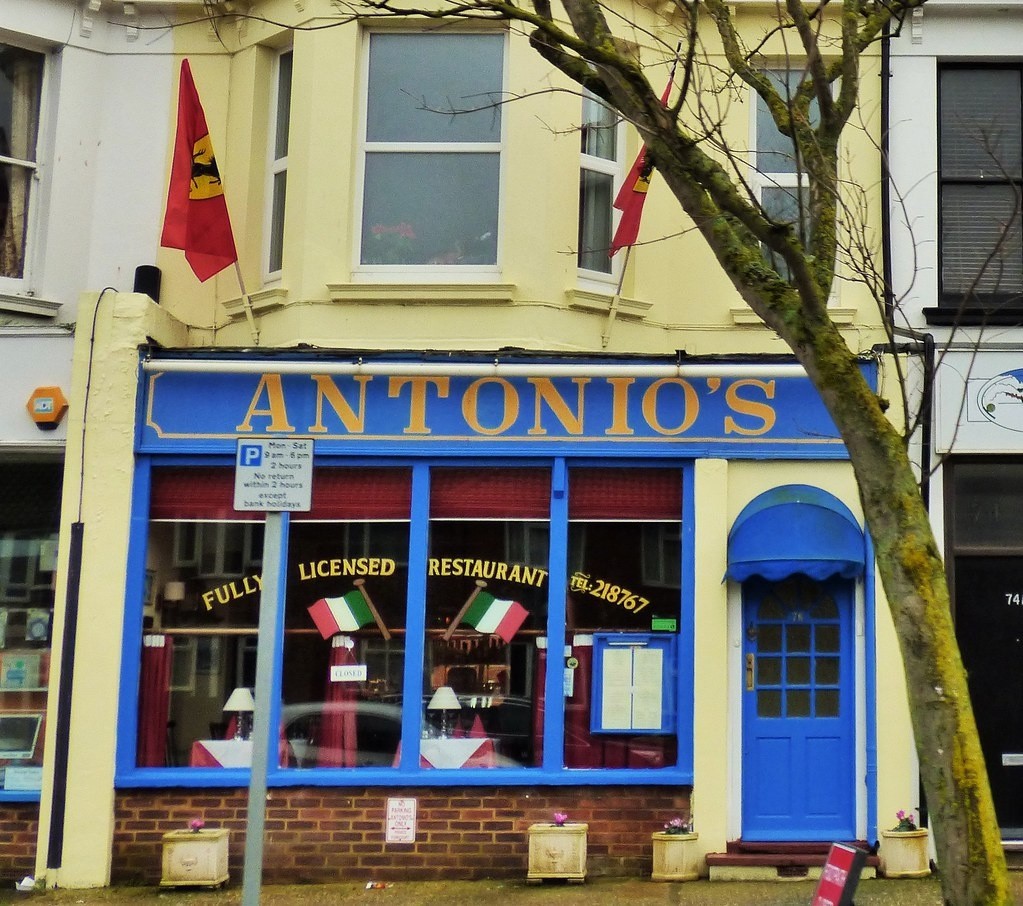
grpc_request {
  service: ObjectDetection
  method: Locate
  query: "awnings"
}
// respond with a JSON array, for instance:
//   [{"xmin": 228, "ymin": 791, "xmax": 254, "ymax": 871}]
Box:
[{"xmin": 727, "ymin": 485, "xmax": 866, "ymax": 583}]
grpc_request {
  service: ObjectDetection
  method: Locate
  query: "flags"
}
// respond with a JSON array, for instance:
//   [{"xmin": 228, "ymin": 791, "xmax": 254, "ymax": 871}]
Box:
[
  {"xmin": 607, "ymin": 67, "xmax": 673, "ymax": 256},
  {"xmin": 157, "ymin": 60, "xmax": 240, "ymax": 282}
]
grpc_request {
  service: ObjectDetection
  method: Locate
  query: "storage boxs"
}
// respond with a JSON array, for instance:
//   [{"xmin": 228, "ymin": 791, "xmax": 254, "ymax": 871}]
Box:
[{"xmin": 0, "ymin": 655, "xmax": 40, "ymax": 689}]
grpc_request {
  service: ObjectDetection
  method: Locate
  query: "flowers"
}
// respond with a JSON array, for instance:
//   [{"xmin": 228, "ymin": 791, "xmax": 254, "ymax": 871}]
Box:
[
  {"xmin": 662, "ymin": 818, "xmax": 689, "ymax": 835},
  {"xmin": 554, "ymin": 812, "xmax": 569, "ymax": 826},
  {"xmin": 893, "ymin": 809, "xmax": 917, "ymax": 830},
  {"xmin": 188, "ymin": 818, "xmax": 206, "ymax": 833},
  {"xmin": 369, "ymin": 223, "xmax": 417, "ymax": 264}
]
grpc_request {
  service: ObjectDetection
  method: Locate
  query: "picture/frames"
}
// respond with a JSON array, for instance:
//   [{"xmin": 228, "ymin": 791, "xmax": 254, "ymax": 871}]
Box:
[{"xmin": 143, "ymin": 568, "xmax": 158, "ymax": 606}]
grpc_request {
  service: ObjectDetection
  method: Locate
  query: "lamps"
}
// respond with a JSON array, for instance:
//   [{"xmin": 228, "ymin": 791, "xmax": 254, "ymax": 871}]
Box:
[
  {"xmin": 427, "ymin": 689, "xmax": 462, "ymax": 740},
  {"xmin": 163, "ymin": 581, "xmax": 185, "ymax": 626},
  {"xmin": 223, "ymin": 688, "xmax": 254, "ymax": 739}
]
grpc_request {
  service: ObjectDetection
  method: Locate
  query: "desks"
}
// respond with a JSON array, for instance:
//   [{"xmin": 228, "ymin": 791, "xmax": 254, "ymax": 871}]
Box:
[
  {"xmin": 191, "ymin": 740, "xmax": 290, "ymax": 767},
  {"xmin": 392, "ymin": 739, "xmax": 498, "ymax": 769}
]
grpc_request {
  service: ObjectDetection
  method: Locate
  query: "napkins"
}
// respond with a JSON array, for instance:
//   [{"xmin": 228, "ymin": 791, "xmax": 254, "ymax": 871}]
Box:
[
  {"xmin": 469, "ymin": 713, "xmax": 487, "ymax": 738},
  {"xmin": 454, "ymin": 716, "xmax": 466, "ymax": 739},
  {"xmin": 226, "ymin": 716, "xmax": 237, "ymax": 739}
]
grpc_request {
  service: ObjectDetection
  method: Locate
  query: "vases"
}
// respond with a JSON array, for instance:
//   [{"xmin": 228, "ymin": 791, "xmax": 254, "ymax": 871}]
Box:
[
  {"xmin": 526, "ymin": 823, "xmax": 588, "ymax": 884},
  {"xmin": 651, "ymin": 832, "xmax": 700, "ymax": 884},
  {"xmin": 880, "ymin": 828, "xmax": 933, "ymax": 879},
  {"xmin": 159, "ymin": 829, "xmax": 230, "ymax": 891}
]
